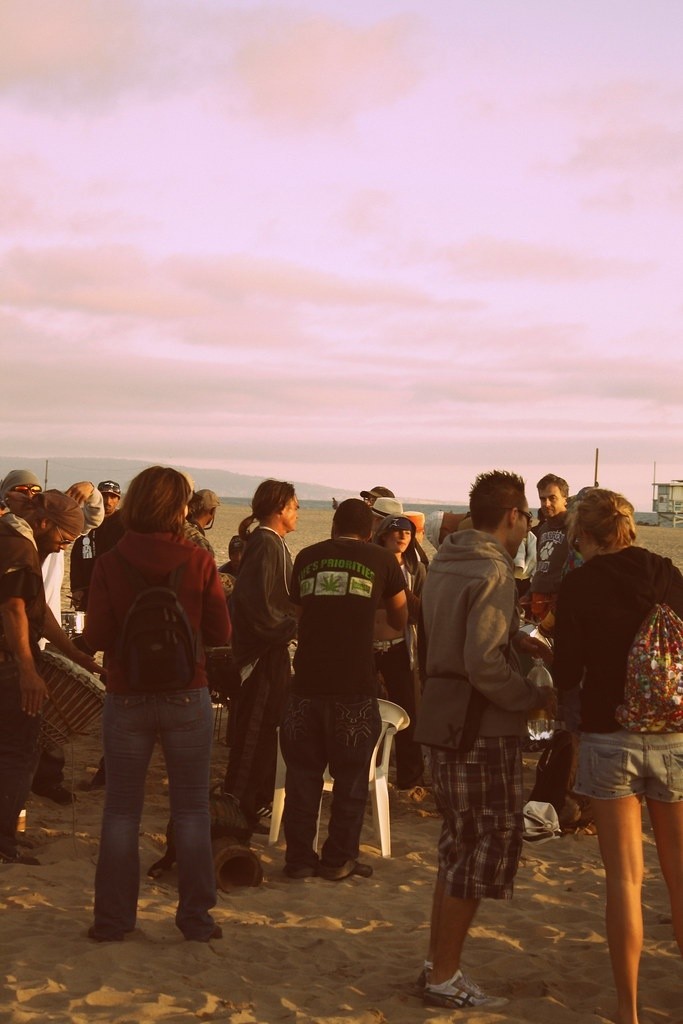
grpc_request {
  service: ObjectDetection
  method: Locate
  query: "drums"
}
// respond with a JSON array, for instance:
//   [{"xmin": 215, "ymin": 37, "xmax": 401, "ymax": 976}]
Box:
[
  {"xmin": 60, "ymin": 610, "xmax": 86, "ymax": 636},
  {"xmin": 166, "ymin": 794, "xmax": 264, "ymax": 894},
  {"xmin": 37, "ymin": 649, "xmax": 108, "ymax": 753}
]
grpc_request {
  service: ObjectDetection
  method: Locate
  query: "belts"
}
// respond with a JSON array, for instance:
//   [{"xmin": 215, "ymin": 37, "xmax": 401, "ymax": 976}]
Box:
[{"xmin": 374, "ymin": 638, "xmax": 404, "ymax": 652}]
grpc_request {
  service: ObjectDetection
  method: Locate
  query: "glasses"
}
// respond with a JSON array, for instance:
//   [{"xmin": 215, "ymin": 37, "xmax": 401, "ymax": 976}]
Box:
[
  {"xmin": 56, "ymin": 525, "xmax": 73, "ymax": 545},
  {"xmin": 12, "ymin": 485, "xmax": 42, "ymax": 496},
  {"xmin": 204, "ymin": 516, "xmax": 214, "ymax": 530},
  {"xmin": 501, "ymin": 507, "xmax": 533, "ymax": 526},
  {"xmin": 364, "ymin": 497, "xmax": 376, "ymax": 504}
]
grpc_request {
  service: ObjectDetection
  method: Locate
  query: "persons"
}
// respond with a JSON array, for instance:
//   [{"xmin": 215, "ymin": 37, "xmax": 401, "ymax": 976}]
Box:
[
  {"xmin": 552, "ymin": 490, "xmax": 683, "ymax": 1024},
  {"xmin": 78, "ymin": 466, "xmax": 230, "ymax": 943},
  {"xmin": 416, "ymin": 471, "xmax": 561, "ymax": 1006},
  {"xmin": 0, "ymin": 466, "xmax": 599, "ymax": 878}
]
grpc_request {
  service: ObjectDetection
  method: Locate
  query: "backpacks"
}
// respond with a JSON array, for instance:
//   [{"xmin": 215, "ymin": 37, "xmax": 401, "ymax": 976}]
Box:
[
  {"xmin": 584, "ymin": 558, "xmax": 683, "ymax": 735},
  {"xmin": 120, "ymin": 554, "xmax": 197, "ymax": 694},
  {"xmin": 529, "ymin": 729, "xmax": 596, "ymax": 829}
]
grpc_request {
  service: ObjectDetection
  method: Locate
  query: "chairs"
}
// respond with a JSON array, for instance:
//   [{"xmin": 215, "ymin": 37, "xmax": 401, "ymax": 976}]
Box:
[{"xmin": 268, "ymin": 701, "xmax": 411, "ymax": 859}]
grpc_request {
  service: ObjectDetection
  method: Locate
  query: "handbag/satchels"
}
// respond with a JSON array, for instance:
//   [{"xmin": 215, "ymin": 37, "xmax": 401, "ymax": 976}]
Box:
[
  {"xmin": 521, "ymin": 801, "xmax": 562, "ymax": 843},
  {"xmin": 147, "ymin": 784, "xmax": 254, "ymax": 879}
]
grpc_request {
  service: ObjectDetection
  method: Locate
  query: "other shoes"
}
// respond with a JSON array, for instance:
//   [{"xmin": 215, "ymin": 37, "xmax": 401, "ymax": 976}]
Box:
[
  {"xmin": 286, "ymin": 858, "xmax": 373, "ymax": 882},
  {"xmin": 0, "ymin": 845, "xmax": 21, "ymax": 860},
  {"xmin": 13, "ymin": 838, "xmax": 34, "ymax": 850},
  {"xmin": 89, "ymin": 925, "xmax": 122, "ymax": 943},
  {"xmin": 178, "ymin": 921, "xmax": 222, "ymax": 941},
  {"xmin": 31, "ymin": 780, "xmax": 76, "ymax": 806}
]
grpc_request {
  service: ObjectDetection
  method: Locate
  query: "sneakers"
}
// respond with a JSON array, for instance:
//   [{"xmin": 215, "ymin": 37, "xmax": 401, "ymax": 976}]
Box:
[{"xmin": 413, "ymin": 960, "xmax": 510, "ymax": 1009}]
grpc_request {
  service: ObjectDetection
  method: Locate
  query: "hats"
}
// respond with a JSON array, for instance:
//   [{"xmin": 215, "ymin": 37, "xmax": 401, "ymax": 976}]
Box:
[
  {"xmin": 360, "ymin": 486, "xmax": 395, "ymax": 498},
  {"xmin": 403, "ymin": 511, "xmax": 424, "ymax": 532},
  {"xmin": 388, "ymin": 518, "xmax": 412, "ymax": 530},
  {"xmin": 195, "ymin": 490, "xmax": 221, "ymax": 510},
  {"xmin": 369, "ymin": 497, "xmax": 403, "ymax": 519},
  {"xmin": 229, "ymin": 535, "xmax": 246, "ymax": 554},
  {"xmin": 97, "ymin": 480, "xmax": 121, "ymax": 497}
]
